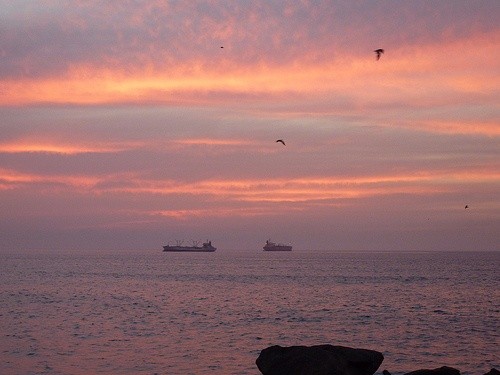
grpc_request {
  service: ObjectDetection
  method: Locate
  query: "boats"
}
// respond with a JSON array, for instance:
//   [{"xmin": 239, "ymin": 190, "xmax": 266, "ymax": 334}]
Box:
[
  {"xmin": 161, "ymin": 239, "xmax": 215, "ymax": 254},
  {"xmin": 262, "ymin": 240, "xmax": 294, "ymax": 252}
]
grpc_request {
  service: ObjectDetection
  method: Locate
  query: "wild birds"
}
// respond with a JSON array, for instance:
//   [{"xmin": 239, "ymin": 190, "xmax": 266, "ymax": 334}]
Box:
[
  {"xmin": 276, "ymin": 140, "xmax": 285, "ymax": 146},
  {"xmin": 373, "ymin": 48, "xmax": 385, "ymax": 61}
]
